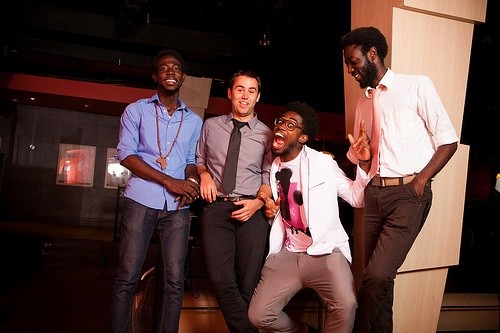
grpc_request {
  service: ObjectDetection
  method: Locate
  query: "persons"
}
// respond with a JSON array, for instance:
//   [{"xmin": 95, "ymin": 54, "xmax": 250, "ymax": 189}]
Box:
[
  {"xmin": 107, "ymin": 170, "xmax": 131, "ymax": 187},
  {"xmin": 195, "ymin": 69, "xmax": 276, "ymax": 333},
  {"xmin": 341, "ymin": 27, "xmax": 459, "ymax": 333},
  {"xmin": 248, "ymin": 103, "xmax": 371, "ymax": 333},
  {"xmin": 111, "ymin": 51, "xmax": 203, "ymax": 333}
]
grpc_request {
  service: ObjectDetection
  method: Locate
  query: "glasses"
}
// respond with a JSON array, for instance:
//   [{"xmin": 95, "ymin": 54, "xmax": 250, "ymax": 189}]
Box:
[{"xmin": 274, "ymin": 117, "xmax": 306, "ymax": 134}]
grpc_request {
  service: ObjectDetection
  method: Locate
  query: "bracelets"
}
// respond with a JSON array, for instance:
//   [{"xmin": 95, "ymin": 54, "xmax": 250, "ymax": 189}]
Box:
[
  {"xmin": 187, "ymin": 178, "xmax": 198, "ymax": 184},
  {"xmin": 358, "ymin": 157, "xmax": 371, "ymax": 162}
]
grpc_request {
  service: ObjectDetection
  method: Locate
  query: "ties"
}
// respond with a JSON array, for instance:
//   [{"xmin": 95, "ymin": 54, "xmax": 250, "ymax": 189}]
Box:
[
  {"xmin": 221, "ymin": 118, "xmax": 247, "ymax": 195},
  {"xmin": 367, "ymin": 85, "xmax": 385, "ymax": 179}
]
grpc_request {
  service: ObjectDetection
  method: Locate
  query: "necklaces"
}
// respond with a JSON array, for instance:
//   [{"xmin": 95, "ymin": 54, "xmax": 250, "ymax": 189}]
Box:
[{"xmin": 154, "ymin": 102, "xmax": 184, "ymax": 170}]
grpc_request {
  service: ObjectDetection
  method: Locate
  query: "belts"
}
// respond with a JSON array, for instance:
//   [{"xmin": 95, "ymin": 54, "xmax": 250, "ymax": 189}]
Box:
[
  {"xmin": 216, "ymin": 196, "xmax": 254, "ymax": 202},
  {"xmin": 371, "ymin": 175, "xmax": 416, "ymax": 186}
]
